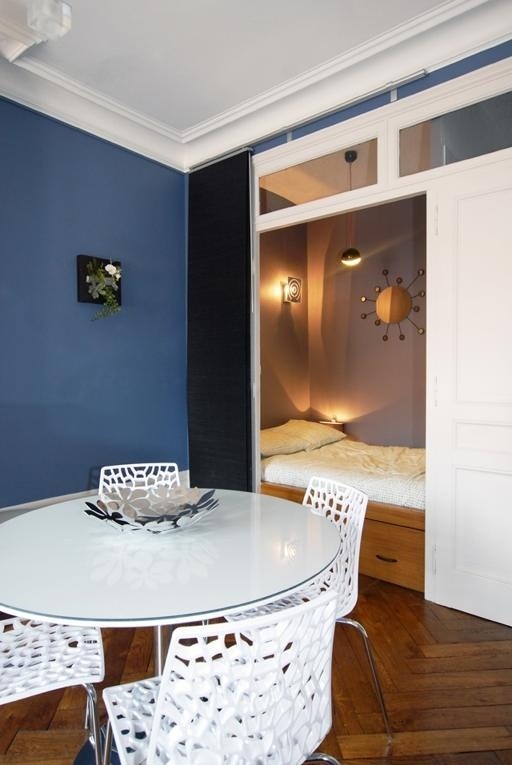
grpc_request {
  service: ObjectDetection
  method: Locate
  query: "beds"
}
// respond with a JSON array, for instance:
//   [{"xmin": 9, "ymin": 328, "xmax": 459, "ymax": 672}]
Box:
[{"xmin": 259, "ymin": 440, "xmax": 425, "ymax": 593}]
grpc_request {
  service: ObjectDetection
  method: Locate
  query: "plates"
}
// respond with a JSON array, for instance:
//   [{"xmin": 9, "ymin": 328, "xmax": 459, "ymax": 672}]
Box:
[{"xmin": 85, "ymin": 487, "xmax": 222, "ymax": 539}]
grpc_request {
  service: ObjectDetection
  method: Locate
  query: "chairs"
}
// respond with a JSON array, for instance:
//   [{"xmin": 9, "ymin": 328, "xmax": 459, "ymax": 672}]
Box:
[
  {"xmin": 98, "ymin": 464, "xmax": 183, "ymax": 496},
  {"xmin": 101, "ymin": 590, "xmax": 341, "ymax": 764},
  {"xmin": 1, "ymin": 617, "xmax": 106, "ymax": 764},
  {"xmin": 202, "ymin": 476, "xmax": 395, "ymax": 743}
]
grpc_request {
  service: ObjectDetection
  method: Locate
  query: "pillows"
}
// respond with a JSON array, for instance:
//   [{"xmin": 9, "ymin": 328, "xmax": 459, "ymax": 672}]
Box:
[{"xmin": 259, "ymin": 418, "xmax": 347, "ymax": 459}]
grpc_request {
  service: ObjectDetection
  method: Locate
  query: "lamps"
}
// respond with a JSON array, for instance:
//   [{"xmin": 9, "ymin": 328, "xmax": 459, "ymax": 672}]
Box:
[
  {"xmin": 341, "ymin": 151, "xmax": 362, "ymax": 266},
  {"xmin": 285, "ymin": 276, "xmax": 303, "ymax": 304}
]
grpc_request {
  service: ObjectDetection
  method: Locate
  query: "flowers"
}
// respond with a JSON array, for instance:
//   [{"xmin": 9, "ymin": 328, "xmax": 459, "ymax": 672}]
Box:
[{"xmin": 88, "ymin": 258, "xmax": 123, "ymax": 322}]
